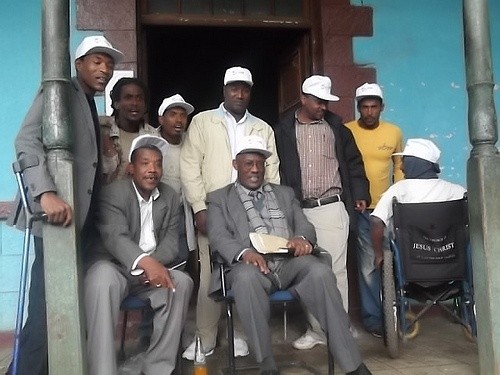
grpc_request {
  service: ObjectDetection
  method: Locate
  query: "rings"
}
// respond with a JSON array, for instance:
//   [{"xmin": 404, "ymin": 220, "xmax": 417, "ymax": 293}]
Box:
[
  {"xmin": 156, "ymin": 283, "xmax": 161, "ymax": 288},
  {"xmin": 305, "ymin": 245, "xmax": 308, "ymax": 248}
]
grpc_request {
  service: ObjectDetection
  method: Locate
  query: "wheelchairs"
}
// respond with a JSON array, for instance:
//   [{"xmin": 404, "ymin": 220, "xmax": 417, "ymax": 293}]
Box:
[{"xmin": 381, "ymin": 193, "xmax": 477, "ymax": 358}]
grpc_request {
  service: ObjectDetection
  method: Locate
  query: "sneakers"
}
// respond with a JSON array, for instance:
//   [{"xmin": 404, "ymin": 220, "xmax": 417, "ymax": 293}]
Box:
[
  {"xmin": 234, "ymin": 338, "xmax": 249, "ymax": 356},
  {"xmin": 182, "ymin": 341, "xmax": 215, "ymax": 360},
  {"xmin": 293, "ymin": 328, "xmax": 327, "ymax": 349}
]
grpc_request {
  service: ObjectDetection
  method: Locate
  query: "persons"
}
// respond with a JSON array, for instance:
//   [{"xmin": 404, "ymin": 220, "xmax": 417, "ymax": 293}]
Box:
[
  {"xmin": 5, "ymin": 36, "xmax": 125, "ymax": 375},
  {"xmin": 369, "ymin": 138, "xmax": 467, "ymax": 299},
  {"xmin": 343, "ymin": 83, "xmax": 405, "ymax": 337},
  {"xmin": 271, "ymin": 75, "xmax": 372, "ymax": 349},
  {"xmin": 140, "ymin": 94, "xmax": 197, "ymax": 354},
  {"xmin": 205, "ymin": 135, "xmax": 373, "ymax": 375},
  {"xmin": 82, "ymin": 134, "xmax": 193, "ymax": 375},
  {"xmin": 179, "ymin": 67, "xmax": 280, "ymax": 361},
  {"xmin": 99, "ymin": 78, "xmax": 155, "ymax": 186}
]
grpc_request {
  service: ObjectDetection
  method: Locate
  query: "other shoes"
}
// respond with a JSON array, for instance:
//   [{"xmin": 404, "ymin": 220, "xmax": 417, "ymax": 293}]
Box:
[
  {"xmin": 373, "ymin": 331, "xmax": 383, "ymax": 338},
  {"xmin": 347, "ymin": 361, "xmax": 372, "ymax": 375}
]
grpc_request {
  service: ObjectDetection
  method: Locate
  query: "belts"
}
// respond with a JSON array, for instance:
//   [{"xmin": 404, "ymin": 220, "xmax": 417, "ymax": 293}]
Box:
[{"xmin": 301, "ymin": 195, "xmax": 342, "ymax": 209}]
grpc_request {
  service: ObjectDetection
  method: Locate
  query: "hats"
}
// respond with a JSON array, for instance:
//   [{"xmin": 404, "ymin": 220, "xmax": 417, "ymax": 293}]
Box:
[
  {"xmin": 129, "ymin": 133, "xmax": 170, "ymax": 163},
  {"xmin": 75, "ymin": 35, "xmax": 125, "ymax": 66},
  {"xmin": 354, "ymin": 83, "xmax": 383, "ymax": 100},
  {"xmin": 235, "ymin": 136, "xmax": 273, "ymax": 161},
  {"xmin": 158, "ymin": 94, "xmax": 194, "ymax": 116},
  {"xmin": 303, "ymin": 76, "xmax": 340, "ymax": 102},
  {"xmin": 224, "ymin": 67, "xmax": 253, "ymax": 86},
  {"xmin": 392, "ymin": 138, "xmax": 441, "ymax": 163}
]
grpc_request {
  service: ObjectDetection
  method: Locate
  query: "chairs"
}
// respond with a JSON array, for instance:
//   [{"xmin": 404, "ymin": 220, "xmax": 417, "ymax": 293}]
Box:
[
  {"xmin": 118, "ymin": 289, "xmax": 184, "ymax": 375},
  {"xmin": 208, "ymin": 240, "xmax": 338, "ymax": 375}
]
